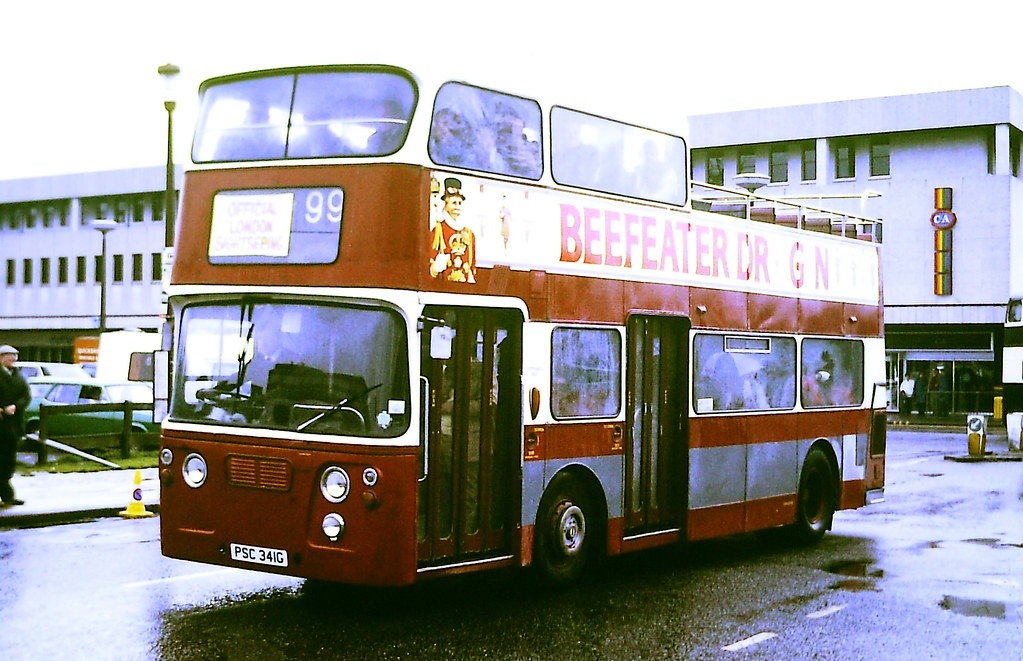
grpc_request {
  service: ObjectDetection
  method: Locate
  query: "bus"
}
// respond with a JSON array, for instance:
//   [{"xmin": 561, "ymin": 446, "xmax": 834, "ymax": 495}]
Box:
[{"xmin": 156, "ymin": 54, "xmax": 889, "ymax": 595}]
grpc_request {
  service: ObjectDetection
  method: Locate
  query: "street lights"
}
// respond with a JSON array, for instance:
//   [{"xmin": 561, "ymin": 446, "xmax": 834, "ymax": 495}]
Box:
[{"xmin": 161, "ymin": 62, "xmax": 179, "ymax": 289}]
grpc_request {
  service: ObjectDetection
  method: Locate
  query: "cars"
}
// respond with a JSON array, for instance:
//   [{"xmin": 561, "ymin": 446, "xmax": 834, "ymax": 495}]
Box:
[{"xmin": 14, "ymin": 359, "xmax": 162, "ymax": 447}]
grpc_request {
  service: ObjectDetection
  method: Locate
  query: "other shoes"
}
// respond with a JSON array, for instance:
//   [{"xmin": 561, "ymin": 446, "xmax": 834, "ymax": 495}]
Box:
[{"xmin": 4, "ymin": 498, "xmax": 26, "ymax": 506}]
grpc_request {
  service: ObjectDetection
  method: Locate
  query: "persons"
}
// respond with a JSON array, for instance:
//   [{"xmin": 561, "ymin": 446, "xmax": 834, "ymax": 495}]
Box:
[
  {"xmin": 428, "ymin": 177, "xmax": 479, "ymax": 285},
  {"xmin": 196, "ymin": 320, "xmax": 306, "ymax": 413},
  {"xmin": 434, "ymin": 335, "xmax": 490, "ymax": 540},
  {"xmin": 710, "ymin": 354, "xmax": 860, "ymax": 409},
  {"xmin": 898, "ymin": 372, "xmax": 915, "ymax": 419},
  {"xmin": 0, "ymin": 345, "xmax": 32, "ymax": 506}
]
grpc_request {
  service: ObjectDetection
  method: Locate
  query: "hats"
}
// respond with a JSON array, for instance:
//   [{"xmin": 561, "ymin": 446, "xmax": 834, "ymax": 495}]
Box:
[{"xmin": 0, "ymin": 345, "xmax": 18, "ymax": 356}]
